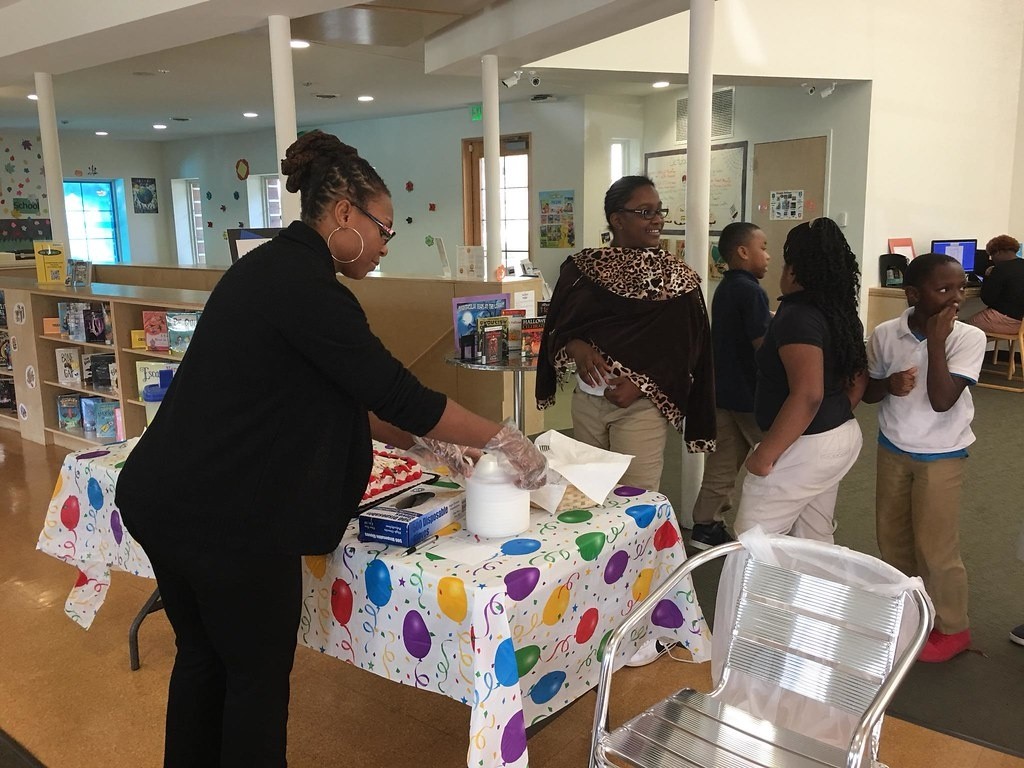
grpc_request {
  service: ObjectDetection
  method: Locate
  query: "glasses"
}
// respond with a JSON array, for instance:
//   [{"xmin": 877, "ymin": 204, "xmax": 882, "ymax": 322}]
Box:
[
  {"xmin": 615, "ymin": 208, "xmax": 668, "ymax": 220},
  {"xmin": 335, "ymin": 200, "xmax": 396, "ymax": 245}
]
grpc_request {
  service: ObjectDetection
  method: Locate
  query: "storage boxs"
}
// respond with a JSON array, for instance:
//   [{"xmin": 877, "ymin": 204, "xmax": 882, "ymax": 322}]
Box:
[
  {"xmin": 135, "ymin": 357, "xmax": 182, "ymax": 402},
  {"xmin": 360, "ymin": 481, "xmax": 465, "ymax": 548}
]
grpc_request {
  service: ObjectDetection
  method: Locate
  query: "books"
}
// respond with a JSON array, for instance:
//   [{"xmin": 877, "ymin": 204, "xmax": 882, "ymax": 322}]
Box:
[
  {"xmin": 33, "ymin": 238, "xmax": 202, "ymax": 444},
  {"xmin": 476, "ymin": 308, "xmax": 546, "ymax": 365}
]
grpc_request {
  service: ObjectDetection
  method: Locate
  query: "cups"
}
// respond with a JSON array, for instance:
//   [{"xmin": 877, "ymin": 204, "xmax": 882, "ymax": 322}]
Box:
[
  {"xmin": 142, "ymin": 383, "xmax": 169, "ymax": 427},
  {"xmin": 464, "ymin": 479, "xmax": 531, "ymax": 539}
]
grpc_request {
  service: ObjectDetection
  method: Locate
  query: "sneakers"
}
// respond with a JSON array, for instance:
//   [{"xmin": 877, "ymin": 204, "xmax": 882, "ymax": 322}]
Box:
[
  {"xmin": 689, "ymin": 520, "xmax": 733, "ymax": 557},
  {"xmin": 918, "ymin": 628, "xmax": 971, "ymax": 663}
]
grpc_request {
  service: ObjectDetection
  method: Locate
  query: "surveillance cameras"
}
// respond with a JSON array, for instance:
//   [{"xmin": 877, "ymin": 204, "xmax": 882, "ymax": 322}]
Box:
[
  {"xmin": 528, "ymin": 76, "xmax": 541, "ymax": 87},
  {"xmin": 501, "ymin": 75, "xmax": 517, "ymax": 88}
]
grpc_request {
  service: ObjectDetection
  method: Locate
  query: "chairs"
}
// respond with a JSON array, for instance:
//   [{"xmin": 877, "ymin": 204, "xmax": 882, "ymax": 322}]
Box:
[
  {"xmin": 976, "ymin": 318, "xmax": 1024, "ymax": 393},
  {"xmin": 587, "ymin": 523, "xmax": 937, "ymax": 767}
]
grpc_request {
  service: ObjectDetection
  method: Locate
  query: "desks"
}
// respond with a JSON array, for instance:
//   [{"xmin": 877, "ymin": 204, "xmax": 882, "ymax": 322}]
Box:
[
  {"xmin": 33, "ymin": 438, "xmax": 714, "ymax": 768},
  {"xmin": 443, "ymin": 348, "xmax": 539, "ymax": 438},
  {"xmin": 866, "ymin": 287, "xmax": 981, "ymax": 339}
]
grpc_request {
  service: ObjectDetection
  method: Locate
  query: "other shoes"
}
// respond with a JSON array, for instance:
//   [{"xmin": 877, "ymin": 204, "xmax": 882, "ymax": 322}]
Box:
[{"xmin": 1009, "ymin": 624, "xmax": 1024, "ymax": 645}]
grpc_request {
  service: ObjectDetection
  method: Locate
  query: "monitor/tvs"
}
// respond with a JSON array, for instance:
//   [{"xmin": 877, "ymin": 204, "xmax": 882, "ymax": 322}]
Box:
[{"xmin": 931, "ymin": 239, "xmax": 976, "ymax": 274}]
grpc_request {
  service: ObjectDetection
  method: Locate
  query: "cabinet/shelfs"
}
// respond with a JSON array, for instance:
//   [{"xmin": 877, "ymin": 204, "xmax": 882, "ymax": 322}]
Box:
[{"xmin": 0, "ymin": 262, "xmax": 545, "ymax": 453}]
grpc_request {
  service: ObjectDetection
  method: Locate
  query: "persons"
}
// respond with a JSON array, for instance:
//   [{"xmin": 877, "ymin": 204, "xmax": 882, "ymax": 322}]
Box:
[
  {"xmin": 113, "ymin": 128, "xmax": 549, "ymax": 767},
  {"xmin": 690, "ymin": 223, "xmax": 776, "ymax": 551},
  {"xmin": 534, "ymin": 176, "xmax": 717, "ymax": 493},
  {"xmin": 733, "ymin": 217, "xmax": 871, "ymax": 543},
  {"xmin": 966, "ymin": 236, "xmax": 1024, "ymax": 339},
  {"xmin": 860, "ymin": 252, "xmax": 987, "ymax": 663}
]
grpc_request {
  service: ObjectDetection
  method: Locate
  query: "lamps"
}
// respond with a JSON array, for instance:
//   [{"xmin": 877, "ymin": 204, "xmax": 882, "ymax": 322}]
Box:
[
  {"xmin": 800, "ymin": 82, "xmax": 816, "ymax": 96},
  {"xmin": 501, "ymin": 70, "xmax": 523, "ymax": 88},
  {"xmin": 820, "ymin": 82, "xmax": 837, "ymax": 98},
  {"xmin": 528, "ymin": 71, "xmax": 541, "ymax": 87}
]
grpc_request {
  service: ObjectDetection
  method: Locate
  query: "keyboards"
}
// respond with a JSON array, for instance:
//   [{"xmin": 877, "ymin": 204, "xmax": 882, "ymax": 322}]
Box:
[{"xmin": 967, "ymin": 281, "xmax": 981, "ymax": 287}]
